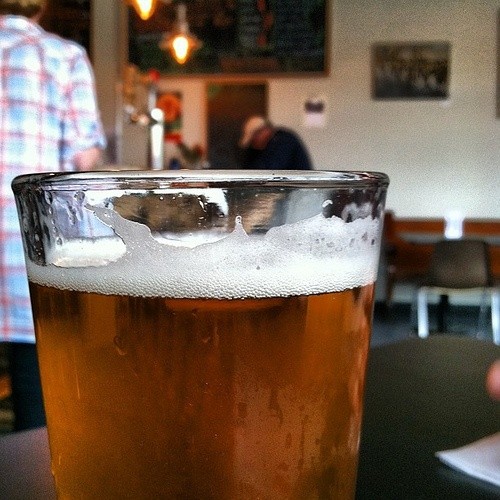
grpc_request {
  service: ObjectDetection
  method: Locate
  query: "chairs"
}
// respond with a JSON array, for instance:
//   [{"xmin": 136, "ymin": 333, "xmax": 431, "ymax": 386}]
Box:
[{"xmin": 382, "ymin": 217, "xmax": 500, "ymax": 339}]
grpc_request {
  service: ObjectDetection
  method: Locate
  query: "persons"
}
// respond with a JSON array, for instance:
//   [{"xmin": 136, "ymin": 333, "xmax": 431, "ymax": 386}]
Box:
[
  {"xmin": 0, "ymin": 0, "xmax": 107, "ymax": 433},
  {"xmin": 239, "ymin": 113, "xmax": 311, "ymax": 170}
]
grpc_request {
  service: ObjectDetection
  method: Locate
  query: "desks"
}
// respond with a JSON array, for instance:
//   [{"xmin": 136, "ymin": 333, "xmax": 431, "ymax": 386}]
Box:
[{"xmin": 0, "ymin": 337, "xmax": 499, "ymax": 499}]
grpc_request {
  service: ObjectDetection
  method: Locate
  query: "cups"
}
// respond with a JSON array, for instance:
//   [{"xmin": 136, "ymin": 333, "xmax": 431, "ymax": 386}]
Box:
[{"xmin": 9, "ymin": 168, "xmax": 391, "ymax": 500}]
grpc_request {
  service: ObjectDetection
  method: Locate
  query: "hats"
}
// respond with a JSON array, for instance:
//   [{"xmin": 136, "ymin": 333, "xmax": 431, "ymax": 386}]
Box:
[{"xmin": 240, "ymin": 116, "xmax": 266, "ymax": 146}]
